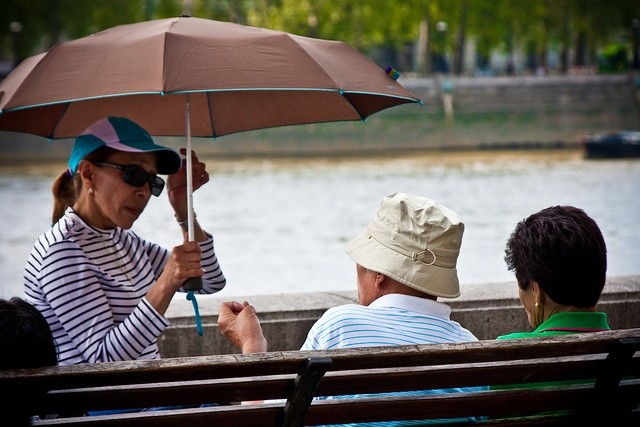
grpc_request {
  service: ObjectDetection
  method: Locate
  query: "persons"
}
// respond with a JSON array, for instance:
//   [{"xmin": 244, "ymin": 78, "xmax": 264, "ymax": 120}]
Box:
[
  {"xmin": 218, "ymin": 192, "xmax": 490, "ymax": 426},
  {"xmin": 490, "ymin": 205, "xmax": 614, "ymax": 426},
  {"xmin": 1, "ymin": 298, "xmax": 62, "ymax": 427},
  {"xmin": 21, "ymin": 116, "xmax": 226, "ymax": 365}
]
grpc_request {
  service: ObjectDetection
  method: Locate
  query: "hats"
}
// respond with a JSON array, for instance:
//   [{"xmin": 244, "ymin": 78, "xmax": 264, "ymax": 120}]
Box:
[
  {"xmin": 66, "ymin": 115, "xmax": 181, "ymax": 175},
  {"xmin": 343, "ymin": 192, "xmax": 466, "ymax": 299}
]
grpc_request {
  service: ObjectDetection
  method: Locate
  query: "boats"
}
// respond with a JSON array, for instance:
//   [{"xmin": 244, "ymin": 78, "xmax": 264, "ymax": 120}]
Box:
[{"xmin": 582, "ymin": 126, "xmax": 640, "ymax": 159}]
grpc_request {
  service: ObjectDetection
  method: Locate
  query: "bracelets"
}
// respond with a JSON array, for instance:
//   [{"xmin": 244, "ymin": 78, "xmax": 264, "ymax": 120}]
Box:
[{"xmin": 180, "ymin": 213, "xmax": 199, "ymax": 226}]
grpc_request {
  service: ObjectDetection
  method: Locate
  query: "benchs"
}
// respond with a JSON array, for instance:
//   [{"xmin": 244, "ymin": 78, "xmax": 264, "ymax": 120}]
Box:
[{"xmin": 0, "ymin": 328, "xmax": 640, "ymax": 427}]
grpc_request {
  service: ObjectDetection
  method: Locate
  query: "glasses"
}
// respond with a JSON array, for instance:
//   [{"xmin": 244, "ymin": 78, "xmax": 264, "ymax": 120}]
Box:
[{"xmin": 92, "ymin": 159, "xmax": 165, "ymax": 197}]
